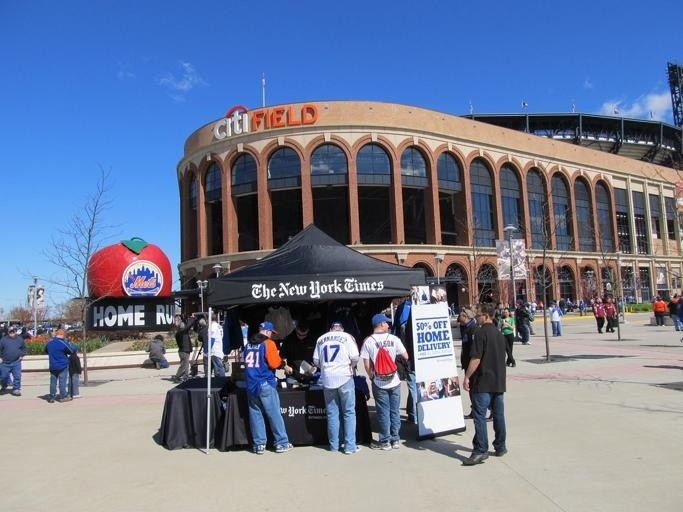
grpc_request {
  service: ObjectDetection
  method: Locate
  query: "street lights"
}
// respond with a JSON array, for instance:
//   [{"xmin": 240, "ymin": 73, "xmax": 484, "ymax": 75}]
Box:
[
  {"xmin": 195, "ymin": 278, "xmax": 210, "ymax": 315},
  {"xmin": 505, "ymin": 222, "xmax": 522, "ymax": 341},
  {"xmin": 503, "ymin": 225, "xmax": 519, "ymax": 308},
  {"xmin": 196, "ymin": 278, "xmax": 208, "ymax": 312},
  {"xmin": 213, "ymin": 263, "xmax": 222, "ymax": 325}
]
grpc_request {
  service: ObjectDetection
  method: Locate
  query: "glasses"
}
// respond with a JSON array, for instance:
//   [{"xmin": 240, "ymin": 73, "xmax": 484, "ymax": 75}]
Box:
[
  {"xmin": 296, "ymin": 333, "xmax": 307, "ymax": 337},
  {"xmin": 476, "ymin": 313, "xmax": 485, "ymax": 317}
]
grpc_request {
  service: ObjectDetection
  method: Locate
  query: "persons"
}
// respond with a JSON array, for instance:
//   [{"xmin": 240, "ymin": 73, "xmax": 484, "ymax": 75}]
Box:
[
  {"xmin": 548, "ymin": 302, "xmax": 562, "ymax": 336},
  {"xmin": 18, "ymin": 327, "xmax": 31, "ymax": 339},
  {"xmin": 461, "ymin": 303, "xmax": 508, "ymax": 466},
  {"xmin": 313, "ymin": 321, "xmax": 361, "ymax": 455},
  {"xmin": 618, "ymin": 295, "xmax": 637, "ymax": 304},
  {"xmin": 203, "ymin": 313, "xmax": 226, "ymax": 377},
  {"xmin": 653, "ymin": 297, "xmax": 667, "ymax": 327},
  {"xmin": 68, "ymin": 350, "xmax": 82, "ymax": 399},
  {"xmin": 579, "ymin": 300, "xmax": 587, "ymax": 316},
  {"xmin": 146, "ymin": 334, "xmax": 170, "ymax": 370},
  {"xmin": 492, "ymin": 303, "xmax": 502, "ymax": 329},
  {"xmin": 242, "ymin": 321, "xmax": 295, "ymax": 455},
  {"xmin": 197, "ymin": 314, "xmax": 212, "ymax": 377},
  {"xmin": 448, "ymin": 305, "xmax": 452, "ymax": 319},
  {"xmin": 279, "ymin": 322, "xmax": 313, "ymax": 389},
  {"xmin": 0, "ymin": 326, "xmax": 27, "ymax": 396},
  {"xmin": 567, "ymin": 297, "xmax": 574, "ymax": 312},
  {"xmin": 361, "ymin": 314, "xmax": 409, "ymax": 450},
  {"xmin": 553, "ymin": 300, "xmax": 563, "ymax": 317},
  {"xmin": 514, "ymin": 298, "xmax": 543, "ymax": 345},
  {"xmin": 438, "ymin": 378, "xmax": 449, "ymax": 399},
  {"xmin": 450, "ymin": 302, "xmax": 455, "ymax": 315},
  {"xmin": 591, "ymin": 298, "xmax": 605, "ymax": 334},
  {"xmin": 44, "ymin": 329, "xmax": 78, "ymax": 404},
  {"xmin": 497, "ymin": 307, "xmax": 516, "ymax": 367},
  {"xmin": 667, "ymin": 296, "xmax": 683, "ymax": 331},
  {"xmin": 171, "ymin": 316, "xmax": 198, "ymax": 383},
  {"xmin": 459, "ymin": 308, "xmax": 494, "ymax": 420},
  {"xmin": 558, "ymin": 298, "xmax": 565, "ymax": 314},
  {"xmin": 428, "ymin": 381, "xmax": 439, "ymax": 401},
  {"xmin": 602, "ymin": 297, "xmax": 616, "ymax": 333},
  {"xmin": 676, "ymin": 290, "xmax": 683, "ymax": 343}
]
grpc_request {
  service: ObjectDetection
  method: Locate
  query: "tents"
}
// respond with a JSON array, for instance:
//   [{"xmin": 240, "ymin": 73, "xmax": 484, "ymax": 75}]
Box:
[{"xmin": 205, "ymin": 223, "xmax": 426, "ymax": 454}]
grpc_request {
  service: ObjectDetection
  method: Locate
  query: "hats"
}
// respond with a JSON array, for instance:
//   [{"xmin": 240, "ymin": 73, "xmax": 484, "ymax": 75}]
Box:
[
  {"xmin": 260, "ymin": 321, "xmax": 278, "ymax": 334},
  {"xmin": 372, "ymin": 314, "xmax": 391, "ymax": 326},
  {"xmin": 658, "ymin": 297, "xmax": 663, "ymax": 300},
  {"xmin": 55, "ymin": 329, "xmax": 65, "ymax": 335},
  {"xmin": 7, "ymin": 326, "xmax": 17, "ymax": 333},
  {"xmin": 550, "ymin": 302, "xmax": 557, "ymax": 304},
  {"xmin": 172, "ymin": 375, "xmax": 180, "ymax": 383},
  {"xmin": 526, "ymin": 303, "xmax": 532, "ymax": 306},
  {"xmin": 332, "ymin": 322, "xmax": 342, "ymax": 329}
]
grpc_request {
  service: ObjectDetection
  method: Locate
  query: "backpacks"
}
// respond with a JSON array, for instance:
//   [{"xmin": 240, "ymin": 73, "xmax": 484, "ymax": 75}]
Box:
[{"xmin": 368, "ymin": 335, "xmax": 398, "ymax": 375}]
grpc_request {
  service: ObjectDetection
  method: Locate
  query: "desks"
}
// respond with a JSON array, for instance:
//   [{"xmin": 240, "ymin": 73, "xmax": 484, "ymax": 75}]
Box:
[{"xmin": 156, "ymin": 376, "xmax": 373, "ymax": 452}]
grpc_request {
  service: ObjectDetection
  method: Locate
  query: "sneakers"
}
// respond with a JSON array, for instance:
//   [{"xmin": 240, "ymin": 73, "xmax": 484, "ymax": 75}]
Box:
[
  {"xmin": 275, "ymin": 443, "xmax": 293, "ymax": 453},
  {"xmin": 369, "ymin": 441, "xmax": 391, "ymax": 450},
  {"xmin": 73, "ymin": 395, "xmax": 81, "ymax": 398},
  {"xmin": 48, "ymin": 398, "xmax": 53, "ymax": 403},
  {"xmin": 606, "ymin": 330, "xmax": 610, "ymax": 332},
  {"xmin": 486, "ymin": 414, "xmax": 493, "ymax": 422},
  {"xmin": 662, "ymin": 325, "xmax": 665, "ymax": 326},
  {"xmin": 496, "ymin": 448, "xmax": 507, "ymax": 456},
  {"xmin": 611, "ymin": 330, "xmax": 615, "ymax": 333},
  {"xmin": 656, "ymin": 324, "xmax": 660, "ymax": 326},
  {"xmin": 344, "ymin": 447, "xmax": 360, "ymax": 454},
  {"xmin": 0, "ymin": 388, "xmax": 7, "ymax": 394},
  {"xmin": 11, "ymin": 389, "xmax": 21, "ymax": 395},
  {"xmin": 155, "ymin": 362, "xmax": 160, "ymax": 369},
  {"xmin": 58, "ymin": 396, "xmax": 72, "ymax": 402},
  {"xmin": 522, "ymin": 342, "xmax": 524, "ymax": 345},
  {"xmin": 392, "ymin": 440, "xmax": 400, "ymax": 449},
  {"xmin": 465, "ymin": 412, "xmax": 473, "ymax": 418},
  {"xmin": 512, "ymin": 363, "xmax": 516, "ymax": 366},
  {"xmin": 524, "ymin": 342, "xmax": 530, "ymax": 345},
  {"xmin": 506, "ymin": 363, "xmax": 510, "ymax": 366},
  {"xmin": 252, "ymin": 446, "xmax": 265, "ymax": 455},
  {"xmin": 463, "ymin": 449, "xmax": 488, "ymax": 465}
]
